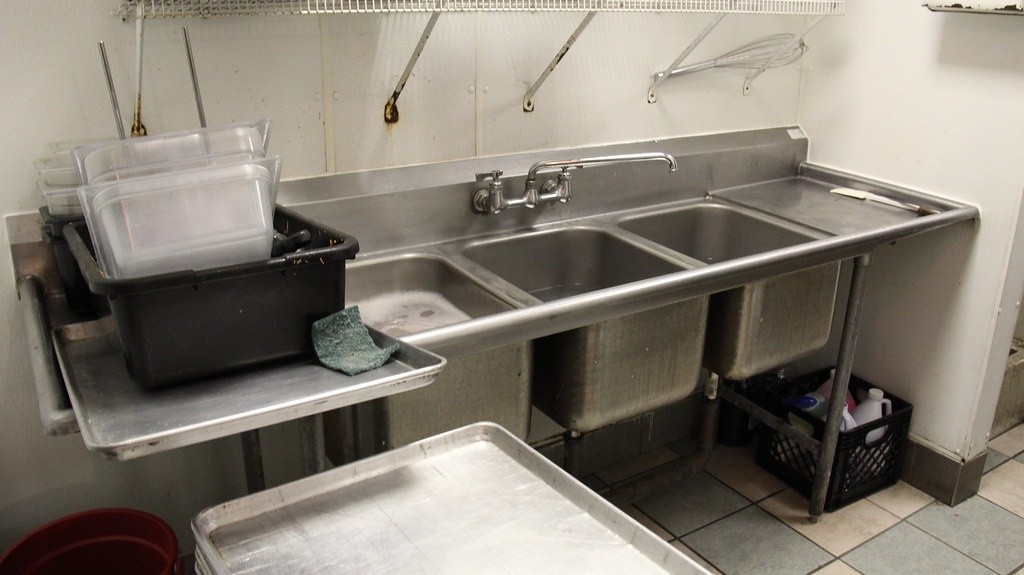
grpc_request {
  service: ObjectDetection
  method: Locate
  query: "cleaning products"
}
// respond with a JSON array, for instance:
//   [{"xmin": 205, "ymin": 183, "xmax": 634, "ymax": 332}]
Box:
[{"xmin": 770, "ymin": 365, "xmax": 895, "ymax": 498}]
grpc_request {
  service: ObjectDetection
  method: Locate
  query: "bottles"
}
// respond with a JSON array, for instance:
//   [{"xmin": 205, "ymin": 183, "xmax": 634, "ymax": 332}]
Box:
[{"xmin": 773, "ymin": 368, "xmax": 894, "ymax": 493}]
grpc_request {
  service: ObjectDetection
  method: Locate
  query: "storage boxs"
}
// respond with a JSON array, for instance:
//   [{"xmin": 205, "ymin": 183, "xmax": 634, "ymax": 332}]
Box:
[
  {"xmin": 26, "ymin": 118, "xmax": 361, "ymax": 398},
  {"xmin": 747, "ymin": 361, "xmax": 914, "ymax": 515}
]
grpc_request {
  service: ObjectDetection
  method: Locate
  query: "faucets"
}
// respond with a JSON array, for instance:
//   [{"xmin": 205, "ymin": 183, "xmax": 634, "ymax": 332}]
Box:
[{"xmin": 525, "ymin": 149, "xmax": 680, "ymax": 209}]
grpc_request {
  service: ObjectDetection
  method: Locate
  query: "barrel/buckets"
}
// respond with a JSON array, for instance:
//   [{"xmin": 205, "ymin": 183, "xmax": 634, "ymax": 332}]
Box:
[{"xmin": 0, "ymin": 509, "xmax": 188, "ymax": 574}]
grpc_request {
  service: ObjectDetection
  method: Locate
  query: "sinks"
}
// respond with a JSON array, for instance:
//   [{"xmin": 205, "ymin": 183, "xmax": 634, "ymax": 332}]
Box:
[
  {"xmin": 456, "ymin": 223, "xmax": 693, "ymax": 309},
  {"xmin": 333, "ymin": 250, "xmax": 526, "ymax": 351},
  {"xmin": 617, "ymin": 202, "xmax": 835, "ymax": 269}
]
